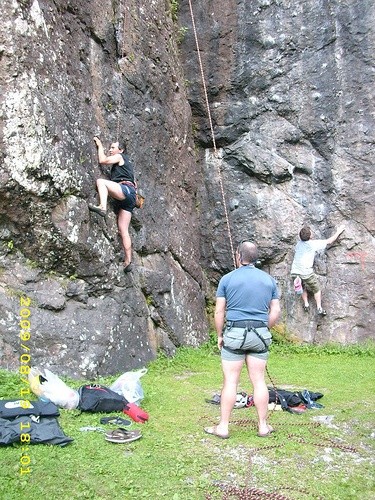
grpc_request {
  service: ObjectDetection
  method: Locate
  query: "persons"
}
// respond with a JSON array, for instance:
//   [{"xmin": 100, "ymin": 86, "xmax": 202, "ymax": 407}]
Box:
[
  {"xmin": 202, "ymin": 239, "xmax": 283, "ymax": 440},
  {"xmin": 89, "ymin": 137, "xmax": 137, "ymax": 271},
  {"xmin": 289, "ymin": 223, "xmax": 346, "ymax": 316}
]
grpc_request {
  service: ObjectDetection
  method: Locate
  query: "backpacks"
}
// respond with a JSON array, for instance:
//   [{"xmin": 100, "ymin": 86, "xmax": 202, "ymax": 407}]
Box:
[{"xmin": 78, "ymin": 384, "xmax": 130, "ymax": 413}]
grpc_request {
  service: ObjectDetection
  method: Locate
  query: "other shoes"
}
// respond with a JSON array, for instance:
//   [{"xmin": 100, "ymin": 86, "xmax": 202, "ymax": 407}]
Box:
[
  {"xmin": 317, "ymin": 308, "xmax": 327, "ymax": 315},
  {"xmin": 125, "ymin": 263, "xmax": 133, "ymax": 273},
  {"xmin": 89, "ymin": 203, "xmax": 107, "ymax": 217},
  {"xmin": 303, "ymin": 303, "xmax": 310, "ymax": 311},
  {"xmin": 100, "ymin": 416, "xmax": 131, "ymax": 427}
]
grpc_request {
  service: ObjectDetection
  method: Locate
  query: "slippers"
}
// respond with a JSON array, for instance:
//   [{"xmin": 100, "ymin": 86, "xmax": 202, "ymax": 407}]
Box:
[
  {"xmin": 105, "ymin": 427, "xmax": 142, "ymax": 437},
  {"xmin": 105, "ymin": 432, "xmax": 141, "ymax": 444},
  {"xmin": 257, "ymin": 424, "xmax": 273, "ymax": 436},
  {"xmin": 204, "ymin": 426, "xmax": 229, "ymax": 438}
]
